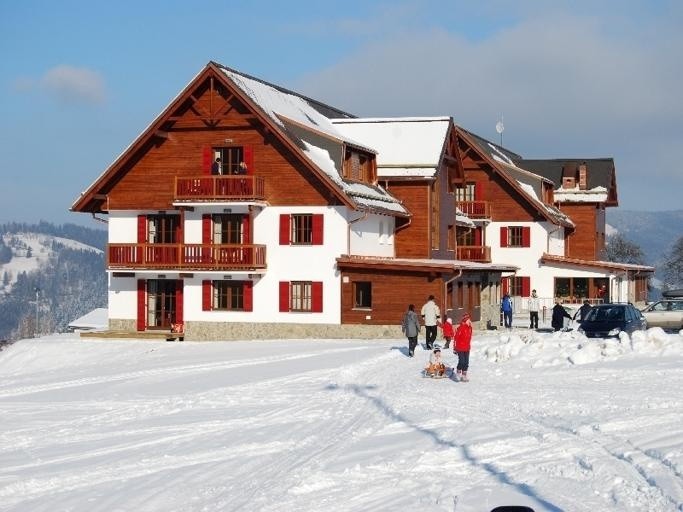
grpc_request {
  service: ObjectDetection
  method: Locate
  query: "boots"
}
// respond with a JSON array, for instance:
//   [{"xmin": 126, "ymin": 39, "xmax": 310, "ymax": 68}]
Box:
[{"xmin": 455, "ymin": 369, "xmax": 469, "ymax": 383}]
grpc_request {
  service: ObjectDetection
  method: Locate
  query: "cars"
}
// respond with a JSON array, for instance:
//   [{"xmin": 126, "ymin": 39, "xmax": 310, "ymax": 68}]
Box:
[{"xmin": 567, "ymin": 300, "xmax": 683, "ymax": 338}]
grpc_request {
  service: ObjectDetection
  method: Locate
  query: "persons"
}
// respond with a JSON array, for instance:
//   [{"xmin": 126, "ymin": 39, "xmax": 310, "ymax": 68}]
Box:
[
  {"xmin": 528, "ymin": 289, "xmax": 540, "ymax": 329},
  {"xmin": 402, "ymin": 304, "xmax": 421, "ymax": 357},
  {"xmin": 501, "ymin": 292, "xmax": 512, "ymax": 328},
  {"xmin": 437, "ymin": 318, "xmax": 454, "ymax": 348},
  {"xmin": 453, "ymin": 315, "xmax": 472, "ymax": 381},
  {"xmin": 421, "ymin": 295, "xmax": 441, "ymax": 350},
  {"xmin": 236, "ymin": 161, "xmax": 247, "ymax": 186},
  {"xmin": 552, "ymin": 298, "xmax": 572, "ymax": 331},
  {"xmin": 211, "ymin": 158, "xmax": 222, "ymax": 175},
  {"xmin": 573, "ymin": 300, "xmax": 592, "ymax": 331}
]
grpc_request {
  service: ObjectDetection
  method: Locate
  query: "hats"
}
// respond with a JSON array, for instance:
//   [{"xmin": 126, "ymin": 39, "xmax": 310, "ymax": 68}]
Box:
[
  {"xmin": 432, "ymin": 344, "xmax": 440, "ymax": 354},
  {"xmin": 445, "ymin": 318, "xmax": 452, "ymax": 324},
  {"xmin": 460, "ymin": 312, "xmax": 471, "ymax": 322}
]
